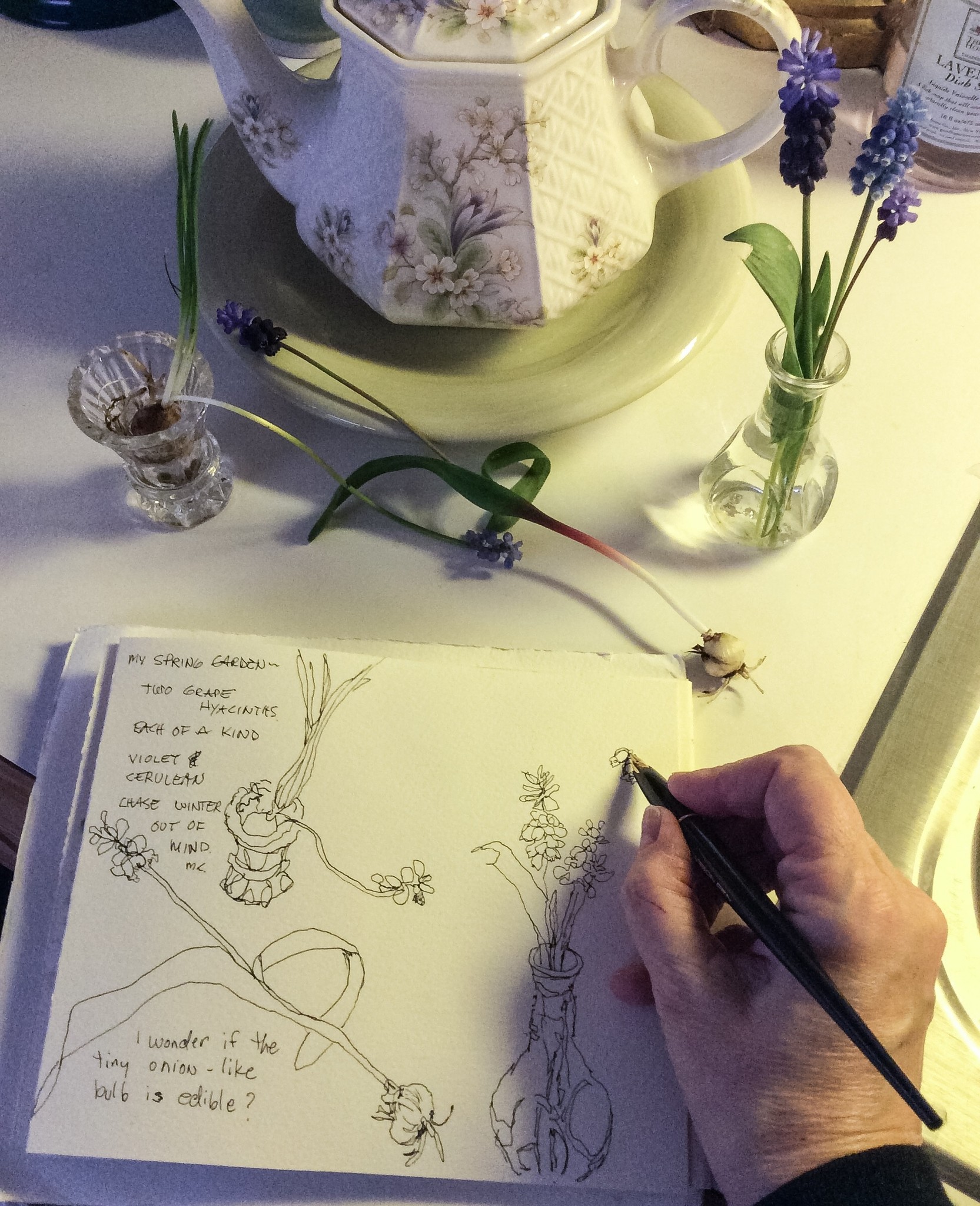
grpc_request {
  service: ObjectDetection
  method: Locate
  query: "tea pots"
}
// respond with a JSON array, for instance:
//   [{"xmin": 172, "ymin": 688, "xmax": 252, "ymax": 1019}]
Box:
[{"xmin": 178, "ymin": 0, "xmax": 806, "ymax": 331}]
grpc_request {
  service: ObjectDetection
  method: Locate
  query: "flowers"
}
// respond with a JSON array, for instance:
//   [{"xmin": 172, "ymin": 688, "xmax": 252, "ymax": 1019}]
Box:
[
  {"xmin": 721, "ymin": 27, "xmax": 939, "ymax": 526},
  {"xmin": 212, "ymin": 304, "xmax": 768, "ymax": 702},
  {"xmin": 134, "ymin": 105, "xmax": 523, "ymax": 569}
]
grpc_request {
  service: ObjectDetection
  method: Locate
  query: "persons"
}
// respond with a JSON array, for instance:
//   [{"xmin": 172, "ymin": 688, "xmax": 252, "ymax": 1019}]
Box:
[{"xmin": 608, "ymin": 744, "xmax": 949, "ymax": 1206}]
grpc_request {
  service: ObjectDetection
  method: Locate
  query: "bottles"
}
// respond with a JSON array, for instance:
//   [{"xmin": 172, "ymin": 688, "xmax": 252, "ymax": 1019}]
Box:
[{"xmin": 872, "ymin": 0, "xmax": 979, "ymax": 194}]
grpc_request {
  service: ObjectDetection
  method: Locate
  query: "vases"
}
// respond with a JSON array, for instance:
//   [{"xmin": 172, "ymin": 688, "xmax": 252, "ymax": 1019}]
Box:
[
  {"xmin": 61, "ymin": 327, "xmax": 234, "ymax": 534},
  {"xmin": 695, "ymin": 330, "xmax": 853, "ymax": 546}
]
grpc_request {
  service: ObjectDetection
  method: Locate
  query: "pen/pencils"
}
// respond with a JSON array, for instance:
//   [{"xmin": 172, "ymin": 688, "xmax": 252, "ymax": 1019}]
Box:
[{"xmin": 626, "ymin": 752, "xmax": 944, "ymax": 1132}]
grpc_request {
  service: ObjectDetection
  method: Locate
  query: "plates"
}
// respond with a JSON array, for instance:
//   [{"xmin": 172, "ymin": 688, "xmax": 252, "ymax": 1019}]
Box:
[{"xmin": 194, "ymin": 47, "xmax": 761, "ymax": 442}]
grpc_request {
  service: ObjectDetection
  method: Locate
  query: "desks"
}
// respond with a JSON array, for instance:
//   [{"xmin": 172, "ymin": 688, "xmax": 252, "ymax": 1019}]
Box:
[{"xmin": 0, "ymin": 10, "xmax": 980, "ymax": 1206}]
